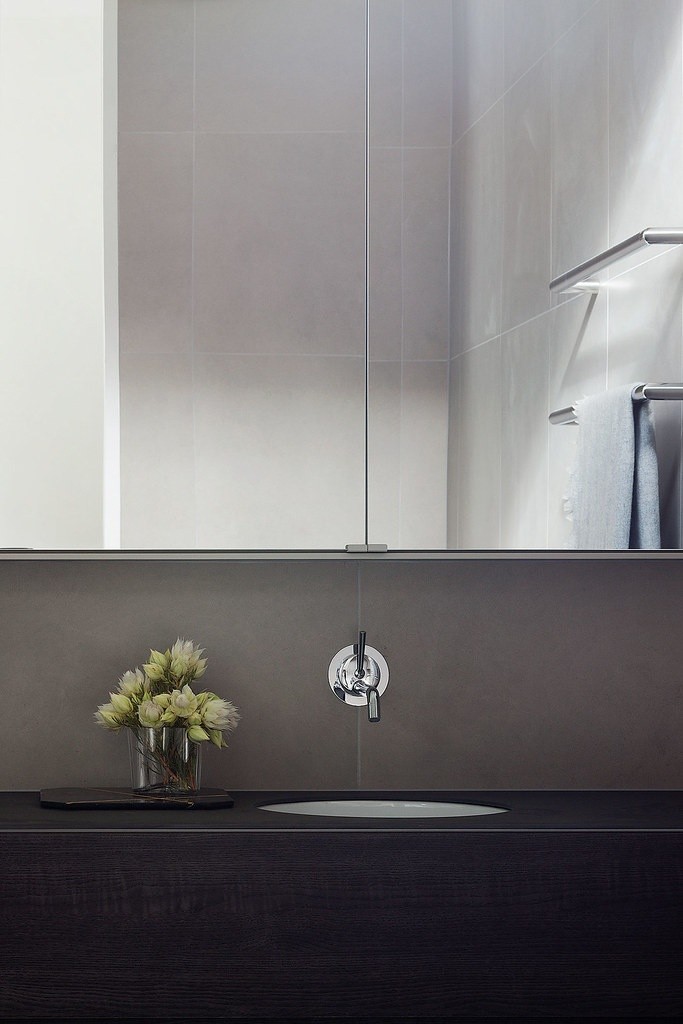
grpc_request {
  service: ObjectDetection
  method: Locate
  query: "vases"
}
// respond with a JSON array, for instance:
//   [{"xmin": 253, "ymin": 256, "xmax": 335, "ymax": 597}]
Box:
[{"xmin": 126, "ymin": 727, "xmax": 203, "ymax": 796}]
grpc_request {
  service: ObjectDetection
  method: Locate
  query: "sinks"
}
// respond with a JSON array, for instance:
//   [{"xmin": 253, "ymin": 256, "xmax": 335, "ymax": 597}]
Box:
[{"xmin": 256, "ymin": 800, "xmax": 510, "ymax": 818}]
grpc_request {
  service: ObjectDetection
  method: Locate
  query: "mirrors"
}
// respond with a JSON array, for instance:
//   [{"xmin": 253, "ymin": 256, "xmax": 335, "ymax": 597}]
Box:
[{"xmin": 0, "ymin": 0, "xmax": 682, "ymax": 551}]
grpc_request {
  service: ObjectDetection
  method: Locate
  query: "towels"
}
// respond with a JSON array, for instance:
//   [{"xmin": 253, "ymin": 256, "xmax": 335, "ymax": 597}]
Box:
[{"xmin": 562, "ymin": 382, "xmax": 661, "ymax": 549}]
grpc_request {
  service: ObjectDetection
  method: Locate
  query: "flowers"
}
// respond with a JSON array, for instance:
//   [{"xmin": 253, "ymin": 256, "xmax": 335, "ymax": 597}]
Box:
[{"xmin": 92, "ymin": 635, "xmax": 242, "ymax": 794}]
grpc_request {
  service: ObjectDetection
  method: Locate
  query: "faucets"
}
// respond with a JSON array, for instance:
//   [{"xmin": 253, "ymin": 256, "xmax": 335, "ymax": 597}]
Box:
[{"xmin": 352, "ymin": 631, "xmax": 381, "ymax": 722}]
